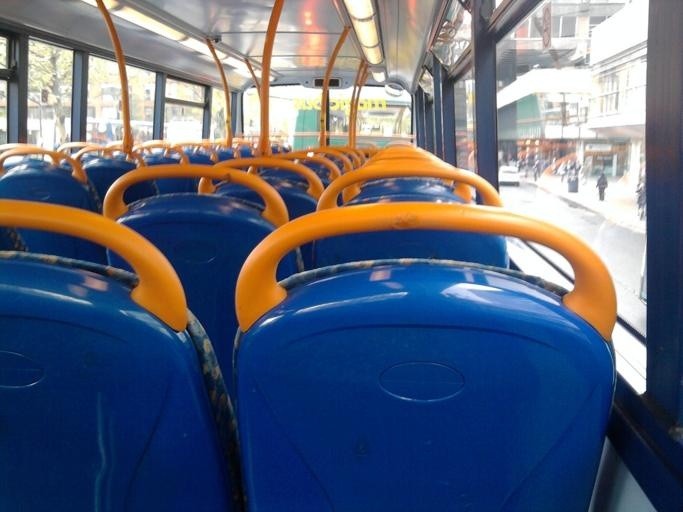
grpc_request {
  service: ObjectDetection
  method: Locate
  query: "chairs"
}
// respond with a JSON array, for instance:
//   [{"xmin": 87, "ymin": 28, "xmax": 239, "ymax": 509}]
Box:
[{"xmin": 0, "ymin": 136, "xmax": 618, "ymax": 512}]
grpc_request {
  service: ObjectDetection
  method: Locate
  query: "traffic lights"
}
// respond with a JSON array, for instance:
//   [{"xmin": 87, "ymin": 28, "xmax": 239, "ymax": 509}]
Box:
[{"xmin": 40, "ymin": 89, "xmax": 48, "ymax": 104}]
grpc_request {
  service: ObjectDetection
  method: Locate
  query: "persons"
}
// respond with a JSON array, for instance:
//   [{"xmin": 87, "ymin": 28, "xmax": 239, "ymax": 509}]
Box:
[
  {"xmin": 636, "ymin": 178, "xmax": 646, "ymax": 220},
  {"xmin": 511, "ymin": 152, "xmax": 581, "ymax": 182},
  {"xmin": 596, "ymin": 173, "xmax": 607, "ymax": 200}
]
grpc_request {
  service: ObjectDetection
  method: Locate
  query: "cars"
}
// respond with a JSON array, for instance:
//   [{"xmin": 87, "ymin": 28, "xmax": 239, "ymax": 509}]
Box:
[{"xmin": 498, "ymin": 165, "xmax": 520, "ymax": 187}]
